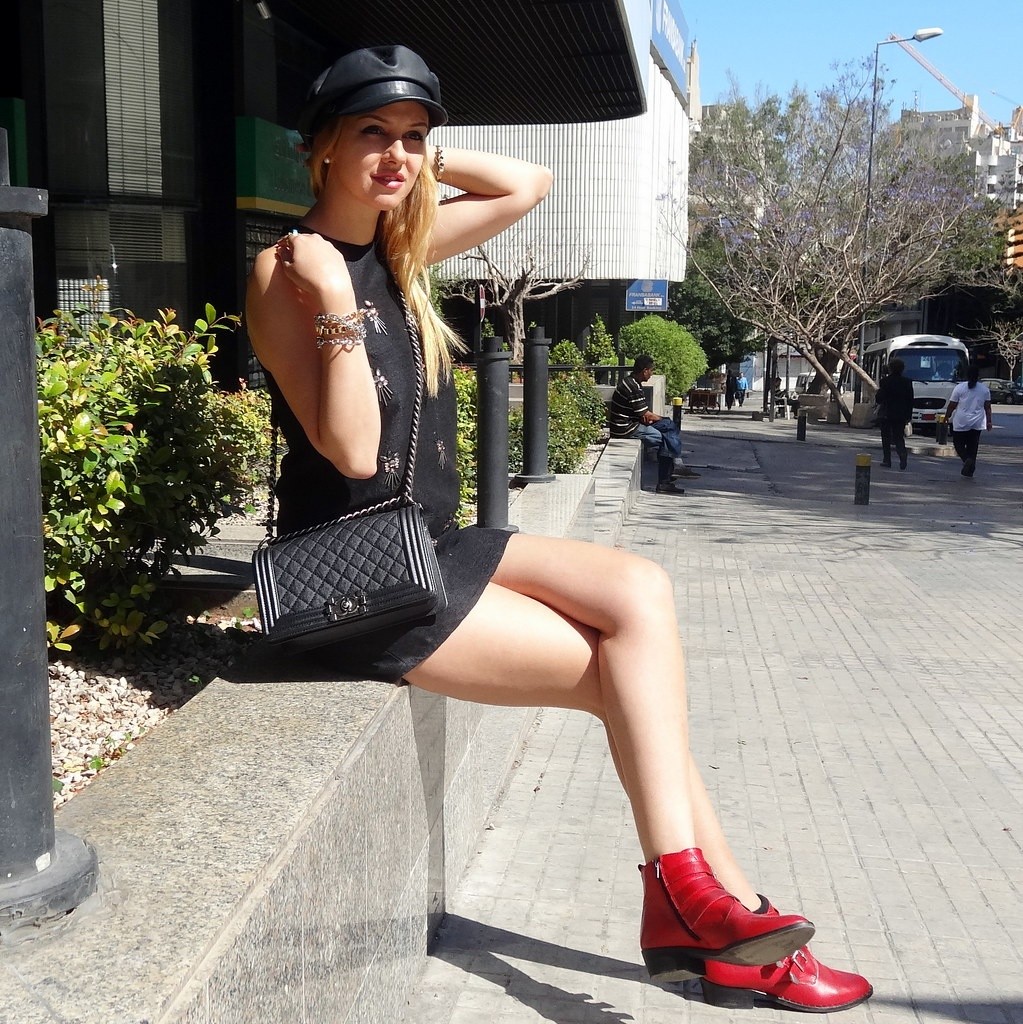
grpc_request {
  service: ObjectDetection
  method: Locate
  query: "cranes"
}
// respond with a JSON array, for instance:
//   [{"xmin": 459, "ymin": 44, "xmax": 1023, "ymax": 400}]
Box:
[
  {"xmin": 987, "ymin": 88, "xmax": 1022, "ymax": 129},
  {"xmin": 888, "ymin": 32, "xmax": 1006, "ymax": 137}
]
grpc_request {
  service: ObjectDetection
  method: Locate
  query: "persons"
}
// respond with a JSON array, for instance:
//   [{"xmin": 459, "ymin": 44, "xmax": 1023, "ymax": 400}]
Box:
[
  {"xmin": 944, "ymin": 366, "xmax": 992, "ymax": 477},
  {"xmin": 875, "ymin": 359, "xmax": 914, "ymax": 470},
  {"xmin": 726, "ymin": 368, "xmax": 748, "ymax": 411},
  {"xmin": 246, "ymin": 44, "xmax": 874, "ymax": 1014},
  {"xmin": 770, "ymin": 378, "xmax": 799, "ymax": 419},
  {"xmin": 610, "ymin": 355, "xmax": 702, "ymax": 494}
]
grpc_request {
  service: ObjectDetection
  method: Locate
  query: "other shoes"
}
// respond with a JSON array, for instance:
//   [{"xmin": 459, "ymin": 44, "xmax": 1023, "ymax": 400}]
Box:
[
  {"xmin": 961, "ymin": 458, "xmax": 973, "ymax": 476},
  {"xmin": 900, "ymin": 465, "xmax": 906, "ymax": 471},
  {"xmin": 968, "ymin": 463, "xmax": 975, "ymax": 477},
  {"xmin": 880, "ymin": 463, "xmax": 891, "ymax": 468}
]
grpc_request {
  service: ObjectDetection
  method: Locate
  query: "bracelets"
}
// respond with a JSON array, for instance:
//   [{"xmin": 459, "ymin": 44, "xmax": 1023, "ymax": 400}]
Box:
[
  {"xmin": 313, "ymin": 312, "xmax": 366, "ymax": 347},
  {"xmin": 436, "ymin": 145, "xmax": 445, "ymax": 181}
]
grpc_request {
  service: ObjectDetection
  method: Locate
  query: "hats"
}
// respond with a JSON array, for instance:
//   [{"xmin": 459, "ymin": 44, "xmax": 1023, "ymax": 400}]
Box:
[{"xmin": 293, "ymin": 44, "xmax": 448, "ymax": 132}]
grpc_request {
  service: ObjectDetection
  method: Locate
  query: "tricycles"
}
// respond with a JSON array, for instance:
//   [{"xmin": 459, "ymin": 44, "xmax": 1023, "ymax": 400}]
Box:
[{"xmin": 682, "ymin": 386, "xmax": 721, "ymax": 416}]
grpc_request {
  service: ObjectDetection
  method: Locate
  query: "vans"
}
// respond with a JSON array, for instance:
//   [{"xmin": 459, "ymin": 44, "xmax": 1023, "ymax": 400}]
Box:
[{"xmin": 794, "ymin": 372, "xmax": 844, "ymax": 397}]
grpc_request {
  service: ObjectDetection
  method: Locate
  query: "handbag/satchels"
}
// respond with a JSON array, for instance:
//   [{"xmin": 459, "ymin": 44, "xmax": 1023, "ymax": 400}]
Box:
[
  {"xmin": 936, "ymin": 419, "xmax": 948, "ymax": 445},
  {"xmin": 870, "ymin": 399, "xmax": 888, "ymax": 422},
  {"xmin": 253, "ymin": 502, "xmax": 448, "ymax": 661}
]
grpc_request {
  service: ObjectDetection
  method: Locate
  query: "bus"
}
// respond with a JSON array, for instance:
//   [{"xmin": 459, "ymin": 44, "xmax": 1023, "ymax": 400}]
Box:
[{"xmin": 860, "ymin": 334, "xmax": 970, "ymax": 437}]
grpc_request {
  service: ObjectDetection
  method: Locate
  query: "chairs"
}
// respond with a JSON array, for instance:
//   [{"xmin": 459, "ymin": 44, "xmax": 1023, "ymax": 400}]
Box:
[
  {"xmin": 936, "ymin": 361, "xmax": 952, "ymax": 379},
  {"xmin": 773, "ymin": 396, "xmax": 797, "ymax": 420}
]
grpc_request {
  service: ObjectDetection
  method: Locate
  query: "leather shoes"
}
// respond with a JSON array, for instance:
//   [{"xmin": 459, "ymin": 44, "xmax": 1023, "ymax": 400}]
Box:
[
  {"xmin": 699, "ymin": 894, "xmax": 873, "ymax": 1012},
  {"xmin": 656, "ymin": 483, "xmax": 684, "ymax": 494},
  {"xmin": 637, "ymin": 848, "xmax": 815, "ymax": 983},
  {"xmin": 658, "ymin": 476, "xmax": 678, "ymax": 482}
]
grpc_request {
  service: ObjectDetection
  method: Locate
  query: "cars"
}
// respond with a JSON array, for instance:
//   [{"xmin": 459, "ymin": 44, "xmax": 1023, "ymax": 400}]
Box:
[
  {"xmin": 981, "ymin": 378, "xmax": 1019, "ymax": 404},
  {"xmin": 1003, "ymin": 380, "xmax": 1015, "ymax": 389},
  {"xmin": 1011, "ymin": 377, "xmax": 1023, "ymax": 405}
]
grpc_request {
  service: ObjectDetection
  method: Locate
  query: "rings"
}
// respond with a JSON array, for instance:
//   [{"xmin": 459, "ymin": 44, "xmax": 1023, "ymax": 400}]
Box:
[{"xmin": 286, "ymin": 233, "xmax": 292, "ymax": 246}]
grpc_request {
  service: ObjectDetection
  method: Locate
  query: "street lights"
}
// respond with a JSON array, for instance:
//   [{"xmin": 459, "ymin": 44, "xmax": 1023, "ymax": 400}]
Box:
[{"xmin": 854, "ymin": 26, "xmax": 945, "ymax": 408}]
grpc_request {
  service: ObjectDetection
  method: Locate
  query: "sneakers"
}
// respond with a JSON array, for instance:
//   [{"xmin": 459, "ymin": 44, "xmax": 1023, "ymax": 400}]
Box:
[{"xmin": 672, "ymin": 468, "xmax": 701, "ymax": 480}]
